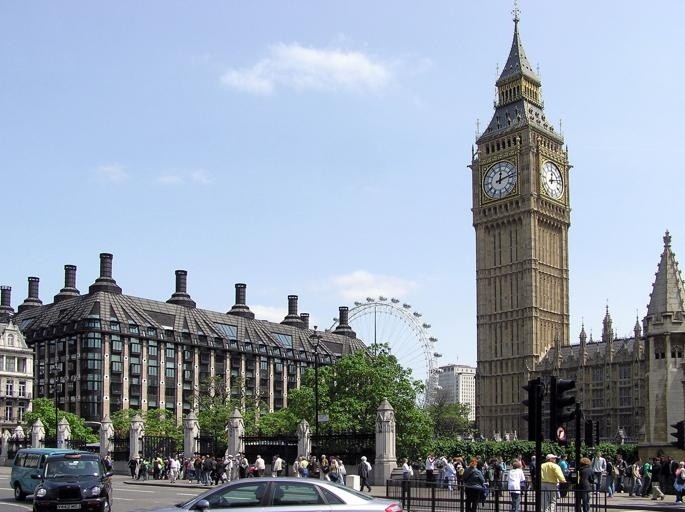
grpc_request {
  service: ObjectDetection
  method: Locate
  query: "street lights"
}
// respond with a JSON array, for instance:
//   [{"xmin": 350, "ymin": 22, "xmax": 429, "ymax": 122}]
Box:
[
  {"xmin": 51, "ymin": 364, "xmax": 61, "ymax": 444},
  {"xmin": 306, "ymin": 325, "xmax": 323, "ymax": 449}
]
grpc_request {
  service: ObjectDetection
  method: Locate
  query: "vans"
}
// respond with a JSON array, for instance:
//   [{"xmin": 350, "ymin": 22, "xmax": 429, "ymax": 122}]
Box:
[{"xmin": 7, "ymin": 445, "xmax": 86, "ymax": 501}]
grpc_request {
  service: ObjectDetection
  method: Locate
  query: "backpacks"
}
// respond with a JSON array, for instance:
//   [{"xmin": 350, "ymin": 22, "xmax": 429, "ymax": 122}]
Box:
[
  {"xmin": 638, "ymin": 466, "xmax": 643, "ymax": 475},
  {"xmin": 625, "ymin": 467, "xmax": 631, "ymax": 477}
]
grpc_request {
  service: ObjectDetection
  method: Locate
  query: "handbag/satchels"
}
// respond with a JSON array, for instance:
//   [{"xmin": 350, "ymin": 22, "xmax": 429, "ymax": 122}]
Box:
[
  {"xmin": 676, "ymin": 472, "xmax": 685, "ymax": 484},
  {"xmin": 611, "ymin": 465, "xmax": 619, "ymax": 478}
]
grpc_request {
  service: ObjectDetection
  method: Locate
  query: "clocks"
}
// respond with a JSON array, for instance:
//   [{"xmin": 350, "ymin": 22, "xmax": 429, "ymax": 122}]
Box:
[
  {"xmin": 482, "ymin": 159, "xmax": 517, "ymax": 200},
  {"xmin": 541, "ymin": 159, "xmax": 564, "ymax": 200}
]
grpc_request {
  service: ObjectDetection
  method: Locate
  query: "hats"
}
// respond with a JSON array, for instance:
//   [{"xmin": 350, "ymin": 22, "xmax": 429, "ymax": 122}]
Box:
[
  {"xmin": 360, "ymin": 456, "xmax": 367, "ymax": 460},
  {"xmin": 545, "ymin": 454, "xmax": 557, "ymax": 461}
]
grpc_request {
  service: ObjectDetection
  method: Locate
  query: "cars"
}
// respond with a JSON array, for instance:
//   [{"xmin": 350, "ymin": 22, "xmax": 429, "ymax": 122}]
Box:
[{"xmin": 133, "ymin": 476, "xmax": 405, "ymax": 512}]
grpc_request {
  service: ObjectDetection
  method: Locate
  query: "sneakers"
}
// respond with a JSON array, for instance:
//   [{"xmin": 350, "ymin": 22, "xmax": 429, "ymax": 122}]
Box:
[
  {"xmin": 661, "ymin": 494, "xmax": 664, "ymax": 501},
  {"xmin": 651, "ymin": 497, "xmax": 657, "ymax": 500}
]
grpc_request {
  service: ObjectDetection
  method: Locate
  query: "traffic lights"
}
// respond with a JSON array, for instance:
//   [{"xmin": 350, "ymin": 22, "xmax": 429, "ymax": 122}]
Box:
[
  {"xmin": 520, "ymin": 377, "xmax": 543, "ymax": 442},
  {"xmin": 547, "ymin": 374, "xmax": 578, "ymax": 439},
  {"xmin": 670, "ymin": 420, "xmax": 685, "ymax": 450}
]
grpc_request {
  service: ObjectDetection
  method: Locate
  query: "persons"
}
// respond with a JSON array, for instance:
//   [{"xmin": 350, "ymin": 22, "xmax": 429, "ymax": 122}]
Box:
[
  {"xmin": 82, "ymin": 462, "xmax": 93, "ymax": 475},
  {"xmin": 293, "ymin": 454, "xmax": 346, "ymax": 486},
  {"xmin": 128, "ymin": 452, "xmax": 265, "ymax": 486},
  {"xmin": 358, "ymin": 456, "xmax": 372, "ymax": 492},
  {"xmin": 272, "ymin": 456, "xmax": 277, "ymax": 477},
  {"xmin": 255, "ymin": 486, "xmax": 266, "ymax": 507},
  {"xmin": 273, "ymin": 454, "xmax": 286, "ymax": 477},
  {"xmin": 396, "ymin": 449, "xmax": 685, "ymax": 512},
  {"xmin": 104, "ymin": 453, "xmax": 111, "ymax": 473}
]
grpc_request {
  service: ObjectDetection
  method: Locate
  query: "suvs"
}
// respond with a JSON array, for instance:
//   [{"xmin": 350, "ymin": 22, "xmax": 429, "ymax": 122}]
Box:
[{"xmin": 30, "ymin": 452, "xmax": 113, "ymax": 511}]
